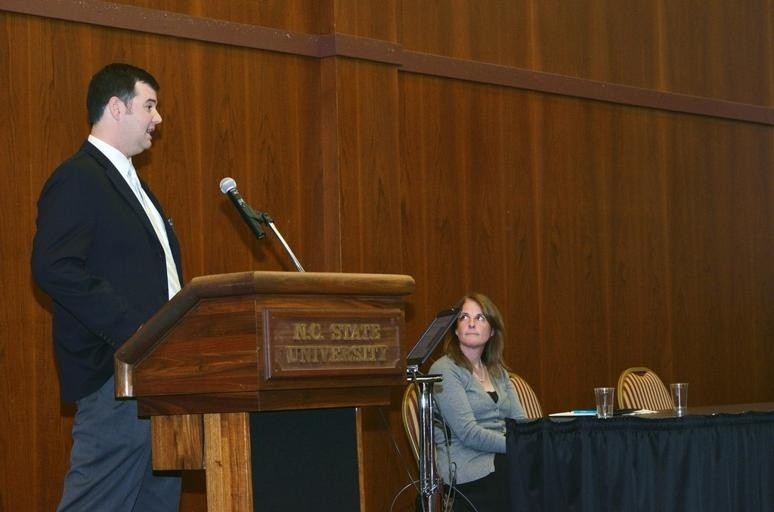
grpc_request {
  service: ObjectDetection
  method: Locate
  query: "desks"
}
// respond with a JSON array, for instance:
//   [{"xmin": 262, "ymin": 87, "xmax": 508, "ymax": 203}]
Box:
[{"xmin": 443, "ymin": 402, "xmax": 774, "ymax": 512}]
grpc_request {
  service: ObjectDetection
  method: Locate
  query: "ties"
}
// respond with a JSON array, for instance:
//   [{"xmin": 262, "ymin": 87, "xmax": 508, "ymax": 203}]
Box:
[{"xmin": 129, "ymin": 174, "xmax": 183, "ymax": 302}]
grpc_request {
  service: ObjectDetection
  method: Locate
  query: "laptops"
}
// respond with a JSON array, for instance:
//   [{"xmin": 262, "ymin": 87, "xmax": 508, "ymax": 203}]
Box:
[{"xmin": 404, "ymin": 307, "xmax": 463, "ymax": 376}]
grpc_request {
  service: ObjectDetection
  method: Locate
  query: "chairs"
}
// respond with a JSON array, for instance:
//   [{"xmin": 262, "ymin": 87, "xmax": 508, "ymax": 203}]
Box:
[
  {"xmin": 618, "ymin": 367, "xmax": 673, "ymax": 410},
  {"xmin": 508, "ymin": 372, "xmax": 544, "ymax": 419},
  {"xmin": 401, "ymin": 382, "xmax": 420, "ymax": 467}
]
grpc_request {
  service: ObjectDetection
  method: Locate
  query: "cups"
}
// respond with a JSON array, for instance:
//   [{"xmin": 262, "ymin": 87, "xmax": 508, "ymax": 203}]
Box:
[
  {"xmin": 669, "ymin": 383, "xmax": 691, "ymax": 411},
  {"xmin": 593, "ymin": 387, "xmax": 616, "ymax": 418}
]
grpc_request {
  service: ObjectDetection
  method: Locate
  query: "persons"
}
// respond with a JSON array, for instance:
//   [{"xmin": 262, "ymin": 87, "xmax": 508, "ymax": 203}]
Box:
[
  {"xmin": 424, "ymin": 294, "xmax": 528, "ymax": 512},
  {"xmin": 29, "ymin": 62, "xmax": 191, "ymax": 512}
]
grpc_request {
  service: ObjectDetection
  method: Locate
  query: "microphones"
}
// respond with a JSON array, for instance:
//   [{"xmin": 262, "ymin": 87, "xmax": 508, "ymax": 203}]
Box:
[{"xmin": 219, "ymin": 178, "xmax": 266, "ymax": 241}]
